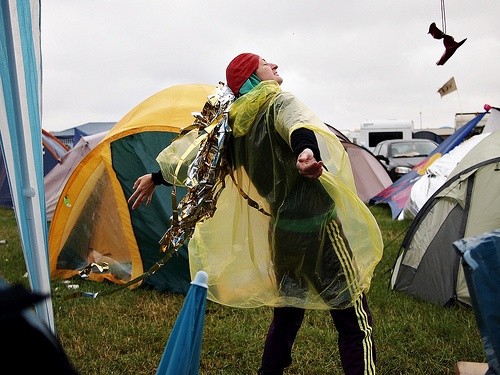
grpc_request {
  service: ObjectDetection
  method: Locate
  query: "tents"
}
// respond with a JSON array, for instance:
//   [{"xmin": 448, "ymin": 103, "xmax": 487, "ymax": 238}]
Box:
[
  {"xmin": 315, "ymin": 122, "xmax": 394, "ymax": 207},
  {"xmin": 0, "ymin": 121, "xmax": 121, "ymax": 221},
  {"xmin": 48, "ymin": 81, "xmax": 274, "ymax": 296},
  {"xmin": 368, "ymin": 106, "xmax": 500, "ymax": 311}
]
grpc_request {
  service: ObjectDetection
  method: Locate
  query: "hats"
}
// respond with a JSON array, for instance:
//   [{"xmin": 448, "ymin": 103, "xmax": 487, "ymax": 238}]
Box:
[{"xmin": 226, "ymin": 54, "xmax": 260, "ymax": 96}]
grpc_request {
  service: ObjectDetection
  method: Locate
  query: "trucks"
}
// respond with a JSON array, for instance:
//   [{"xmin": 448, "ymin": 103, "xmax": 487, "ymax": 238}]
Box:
[{"xmin": 341, "ymin": 111, "xmax": 491, "ymax": 155}]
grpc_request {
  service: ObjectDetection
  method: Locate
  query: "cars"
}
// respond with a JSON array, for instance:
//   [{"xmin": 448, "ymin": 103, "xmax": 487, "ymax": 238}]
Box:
[{"xmin": 371, "ymin": 138, "xmax": 440, "ymax": 183}]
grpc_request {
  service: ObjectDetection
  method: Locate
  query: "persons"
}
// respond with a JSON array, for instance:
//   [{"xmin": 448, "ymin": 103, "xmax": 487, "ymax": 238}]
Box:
[{"xmin": 128, "ymin": 53, "xmax": 377, "ymax": 375}]
